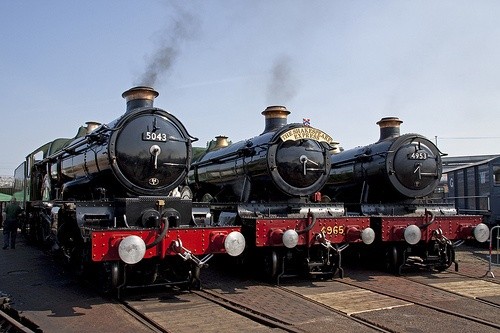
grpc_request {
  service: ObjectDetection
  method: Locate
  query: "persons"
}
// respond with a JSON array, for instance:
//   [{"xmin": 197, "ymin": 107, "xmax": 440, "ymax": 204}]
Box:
[{"xmin": 2, "ymin": 197, "xmax": 21, "ymax": 249}]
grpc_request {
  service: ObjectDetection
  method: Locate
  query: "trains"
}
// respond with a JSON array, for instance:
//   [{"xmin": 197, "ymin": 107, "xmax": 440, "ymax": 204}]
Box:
[
  {"xmin": 12, "ymin": 85, "xmax": 246, "ymax": 292},
  {"xmin": 185, "ymin": 105, "xmax": 374, "ymax": 283},
  {"xmin": 322, "ymin": 116, "xmax": 490, "ymax": 275}
]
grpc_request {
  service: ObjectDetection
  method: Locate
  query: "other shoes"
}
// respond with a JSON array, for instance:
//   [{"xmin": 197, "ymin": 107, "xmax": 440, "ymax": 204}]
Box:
[
  {"xmin": 12, "ymin": 247, "xmax": 16, "ymax": 249},
  {"xmin": 2, "ymin": 245, "xmax": 9, "ymax": 250}
]
